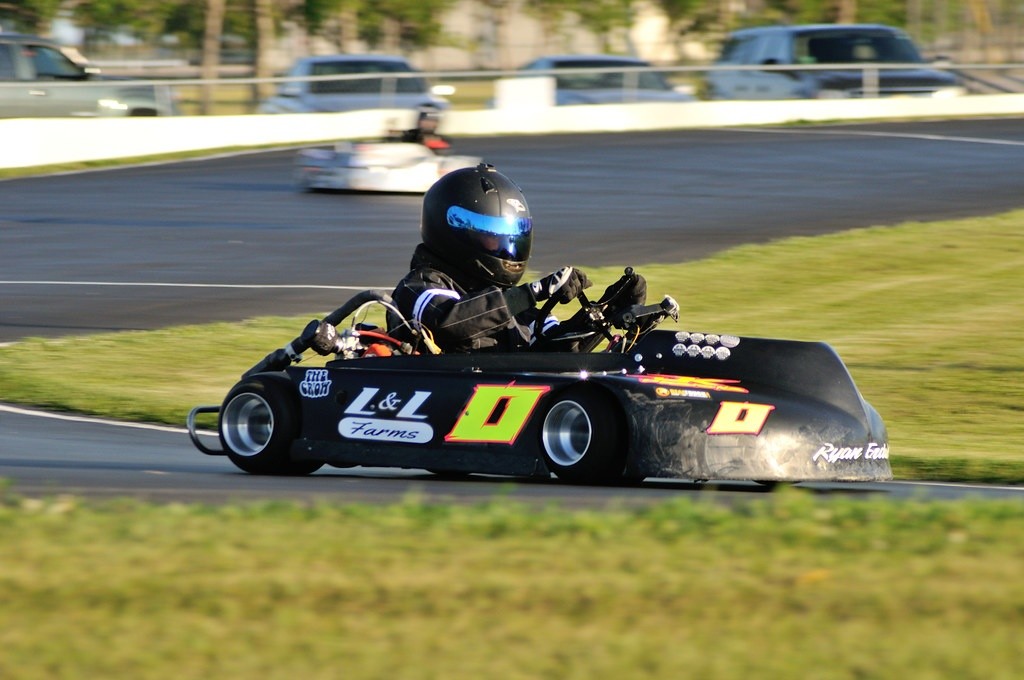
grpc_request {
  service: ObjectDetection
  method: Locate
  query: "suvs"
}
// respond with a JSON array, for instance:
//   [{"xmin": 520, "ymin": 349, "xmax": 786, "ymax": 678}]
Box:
[{"xmin": 702, "ymin": 22, "xmax": 966, "ymax": 101}]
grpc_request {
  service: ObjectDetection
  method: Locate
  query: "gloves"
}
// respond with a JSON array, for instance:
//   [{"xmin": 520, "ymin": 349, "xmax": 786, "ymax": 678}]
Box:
[
  {"xmin": 597, "ymin": 267, "xmax": 649, "ymax": 317},
  {"xmin": 525, "ymin": 266, "xmax": 592, "ymax": 307}
]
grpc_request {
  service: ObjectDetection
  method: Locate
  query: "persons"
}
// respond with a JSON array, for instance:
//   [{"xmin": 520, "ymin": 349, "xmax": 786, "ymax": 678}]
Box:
[
  {"xmin": 387, "ymin": 163, "xmax": 648, "ymax": 354},
  {"xmin": 382, "ymin": 103, "xmax": 453, "ymax": 153}
]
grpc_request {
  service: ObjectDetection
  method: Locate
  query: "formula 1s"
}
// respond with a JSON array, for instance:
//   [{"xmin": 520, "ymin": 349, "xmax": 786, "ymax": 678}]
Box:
[
  {"xmin": 184, "ymin": 267, "xmax": 894, "ymax": 486},
  {"xmin": 290, "ymin": 134, "xmax": 489, "ymax": 196}
]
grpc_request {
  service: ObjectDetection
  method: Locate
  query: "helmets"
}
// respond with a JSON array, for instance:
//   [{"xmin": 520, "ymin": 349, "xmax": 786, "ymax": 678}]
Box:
[{"xmin": 420, "ymin": 164, "xmax": 534, "ymax": 286}]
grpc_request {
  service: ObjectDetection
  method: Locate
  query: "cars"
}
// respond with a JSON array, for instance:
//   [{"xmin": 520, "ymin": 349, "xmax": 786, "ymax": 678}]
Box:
[
  {"xmin": 516, "ymin": 55, "xmax": 699, "ymax": 103},
  {"xmin": 2, "ymin": 32, "xmax": 180, "ymax": 118},
  {"xmin": 254, "ymin": 53, "xmax": 452, "ymax": 111}
]
